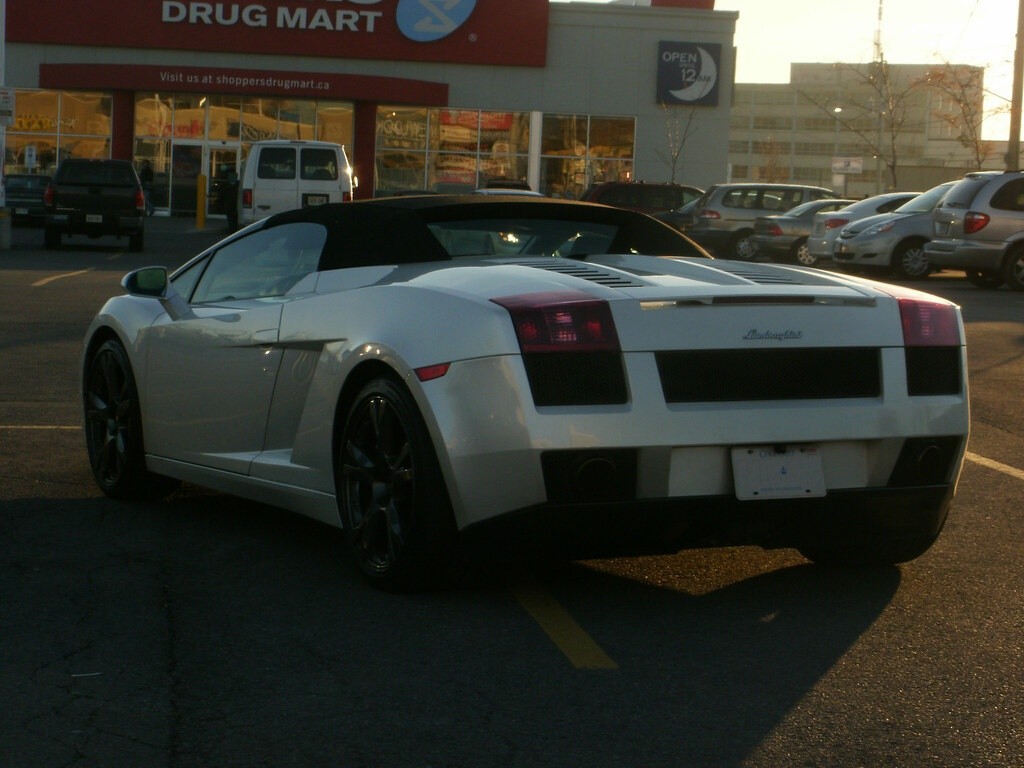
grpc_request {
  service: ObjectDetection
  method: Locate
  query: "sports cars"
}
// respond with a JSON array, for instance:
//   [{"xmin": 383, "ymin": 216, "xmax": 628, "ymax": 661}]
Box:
[{"xmin": 79, "ymin": 191, "xmax": 974, "ymax": 593}]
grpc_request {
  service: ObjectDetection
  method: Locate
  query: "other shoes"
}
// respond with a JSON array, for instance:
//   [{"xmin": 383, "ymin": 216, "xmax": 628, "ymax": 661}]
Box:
[{"xmin": 150, "ymin": 208, "xmax": 156, "ymax": 216}]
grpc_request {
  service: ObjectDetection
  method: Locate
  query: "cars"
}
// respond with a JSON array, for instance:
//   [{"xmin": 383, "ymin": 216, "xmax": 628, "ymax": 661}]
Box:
[
  {"xmin": 44, "ymin": 157, "xmax": 146, "ymax": 250},
  {"xmin": 691, "ymin": 182, "xmax": 842, "ymax": 262},
  {"xmin": 674, "ymin": 194, "xmax": 783, "ymax": 235},
  {"xmin": 4, "ymin": 174, "xmax": 55, "ymax": 227},
  {"xmin": 833, "ymin": 180, "xmax": 963, "ymax": 280},
  {"xmin": 750, "ymin": 199, "xmax": 857, "ymax": 267},
  {"xmin": 806, "ymin": 192, "xmax": 921, "ymax": 258}
]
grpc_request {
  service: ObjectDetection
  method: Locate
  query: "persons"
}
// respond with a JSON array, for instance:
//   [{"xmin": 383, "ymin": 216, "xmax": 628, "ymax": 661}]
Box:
[
  {"xmin": 223, "ymin": 171, "xmax": 242, "ymax": 231},
  {"xmin": 140, "ymin": 159, "xmax": 155, "ymax": 215}
]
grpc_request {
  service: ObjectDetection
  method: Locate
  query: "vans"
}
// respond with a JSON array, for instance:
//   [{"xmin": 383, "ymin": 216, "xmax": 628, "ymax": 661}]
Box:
[{"xmin": 236, "ymin": 139, "xmax": 360, "ymax": 227}]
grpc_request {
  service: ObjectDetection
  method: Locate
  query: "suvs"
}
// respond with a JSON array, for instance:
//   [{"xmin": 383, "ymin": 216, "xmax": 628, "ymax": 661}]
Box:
[
  {"xmin": 577, "ymin": 179, "xmax": 706, "ymax": 211},
  {"xmin": 923, "ymin": 167, "xmax": 1024, "ymax": 291}
]
